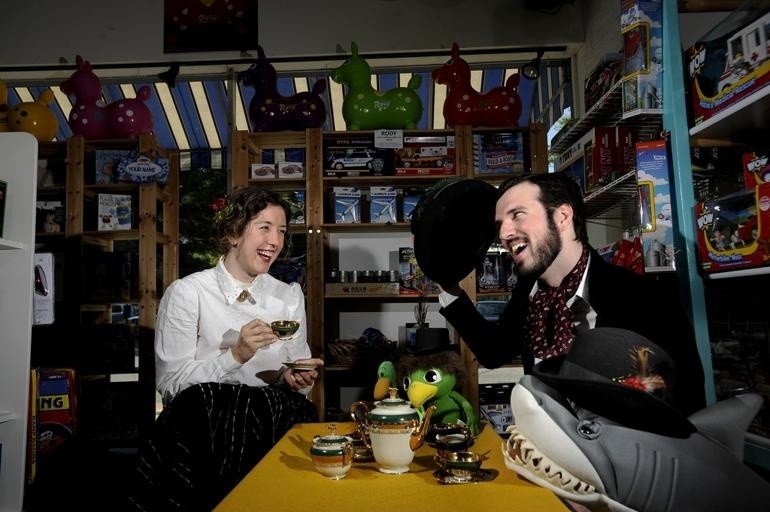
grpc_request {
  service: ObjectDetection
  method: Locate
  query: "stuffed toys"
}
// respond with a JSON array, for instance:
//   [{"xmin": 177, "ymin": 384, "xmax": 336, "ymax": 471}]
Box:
[
  {"xmin": 400, "ymin": 327, "xmax": 482, "ymax": 437},
  {"xmin": 373, "ymin": 360, "xmax": 403, "ymax": 399},
  {"xmin": 501, "ymin": 374, "xmax": 770, "ymax": 512}
]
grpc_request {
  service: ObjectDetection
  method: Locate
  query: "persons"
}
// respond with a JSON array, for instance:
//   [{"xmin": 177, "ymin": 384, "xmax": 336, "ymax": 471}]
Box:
[
  {"xmin": 434, "ymin": 171, "xmax": 692, "ymax": 439},
  {"xmin": 127, "ymin": 184, "xmax": 325, "ymax": 512}
]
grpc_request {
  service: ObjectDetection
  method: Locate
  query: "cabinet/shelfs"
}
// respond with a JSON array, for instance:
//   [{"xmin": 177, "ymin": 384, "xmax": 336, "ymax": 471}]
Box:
[
  {"xmin": 0, "ymin": 131, "xmax": 37, "ymax": 452},
  {"xmin": 32, "ymin": 144, "xmax": 181, "ymax": 327},
  {"xmin": 224, "ymin": 128, "xmax": 543, "ymax": 417},
  {"xmin": 544, "ymin": 0, "xmax": 770, "ymax": 407}
]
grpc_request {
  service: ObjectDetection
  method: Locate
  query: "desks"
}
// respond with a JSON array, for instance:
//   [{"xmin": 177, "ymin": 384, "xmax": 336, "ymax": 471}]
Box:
[{"xmin": 211, "ymin": 420, "xmax": 570, "ymax": 512}]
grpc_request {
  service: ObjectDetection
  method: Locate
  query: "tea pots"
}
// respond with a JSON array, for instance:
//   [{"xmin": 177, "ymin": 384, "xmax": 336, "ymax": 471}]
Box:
[
  {"xmin": 351, "ymin": 387, "xmax": 436, "ymax": 474},
  {"xmin": 310, "ymin": 425, "xmax": 356, "ymax": 480}
]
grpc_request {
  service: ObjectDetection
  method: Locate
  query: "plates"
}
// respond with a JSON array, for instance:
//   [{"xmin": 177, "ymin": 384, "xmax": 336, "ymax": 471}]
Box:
[
  {"xmin": 282, "ymin": 362, "xmax": 317, "ymax": 370},
  {"xmin": 433, "ymin": 468, "xmax": 494, "ymax": 484},
  {"xmin": 353, "ymin": 452, "xmax": 374, "ymax": 462}
]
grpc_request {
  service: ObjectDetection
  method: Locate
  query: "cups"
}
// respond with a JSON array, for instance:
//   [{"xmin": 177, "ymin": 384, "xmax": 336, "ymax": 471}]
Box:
[
  {"xmin": 344, "ymin": 433, "xmax": 371, "ymax": 456},
  {"xmin": 434, "ymin": 451, "xmax": 483, "ymax": 481},
  {"xmin": 271, "ymin": 320, "xmax": 300, "ymax": 340}
]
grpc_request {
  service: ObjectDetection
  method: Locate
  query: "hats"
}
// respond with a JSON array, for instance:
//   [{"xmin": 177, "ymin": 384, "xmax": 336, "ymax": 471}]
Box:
[
  {"xmin": 410, "ymin": 173, "xmax": 500, "ymax": 286},
  {"xmin": 531, "ymin": 326, "xmax": 680, "ymax": 433}
]
